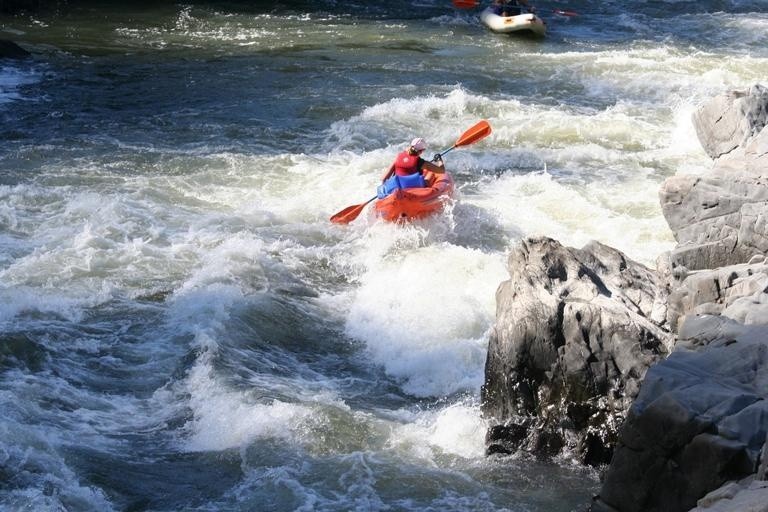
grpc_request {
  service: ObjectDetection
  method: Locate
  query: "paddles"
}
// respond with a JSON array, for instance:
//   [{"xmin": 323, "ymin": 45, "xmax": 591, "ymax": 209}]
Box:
[
  {"xmin": 330, "ymin": 121, "xmax": 490, "ymax": 223},
  {"xmin": 453, "ymin": 0, "xmax": 575, "ymax": 15}
]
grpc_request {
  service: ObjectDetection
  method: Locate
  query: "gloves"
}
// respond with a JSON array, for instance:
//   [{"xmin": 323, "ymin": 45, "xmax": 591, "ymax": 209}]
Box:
[{"xmin": 434, "ymin": 154, "xmax": 440, "ymax": 161}]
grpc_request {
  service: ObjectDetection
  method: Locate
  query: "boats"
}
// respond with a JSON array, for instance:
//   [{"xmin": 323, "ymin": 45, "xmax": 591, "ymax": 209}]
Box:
[
  {"xmin": 374, "ymin": 162, "xmax": 456, "ymax": 226},
  {"xmin": 480, "ymin": 5, "xmax": 548, "ymax": 41}
]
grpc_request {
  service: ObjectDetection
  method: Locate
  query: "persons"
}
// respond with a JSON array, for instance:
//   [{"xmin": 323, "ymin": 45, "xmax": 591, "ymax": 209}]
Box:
[
  {"xmin": 494, "ymin": 1, "xmax": 537, "ymax": 18},
  {"xmin": 382, "ymin": 137, "xmax": 446, "ymax": 188}
]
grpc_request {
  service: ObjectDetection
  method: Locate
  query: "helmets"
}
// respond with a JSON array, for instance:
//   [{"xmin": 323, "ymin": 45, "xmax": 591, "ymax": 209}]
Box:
[{"xmin": 412, "ymin": 138, "xmax": 425, "ymax": 150}]
